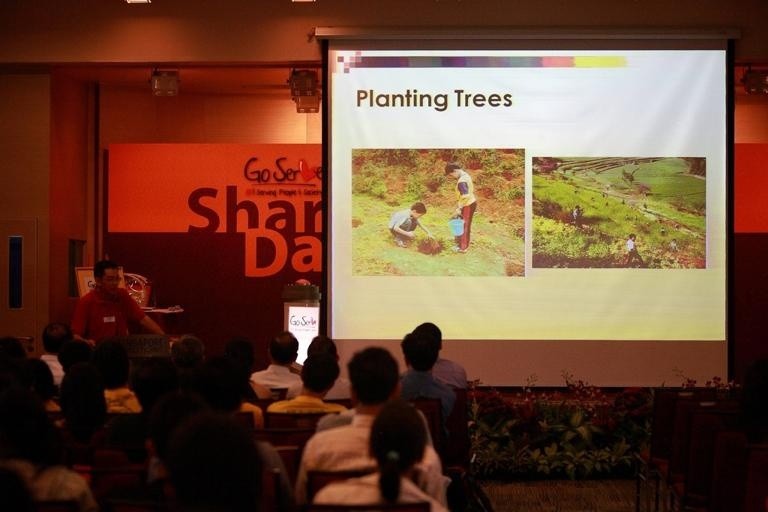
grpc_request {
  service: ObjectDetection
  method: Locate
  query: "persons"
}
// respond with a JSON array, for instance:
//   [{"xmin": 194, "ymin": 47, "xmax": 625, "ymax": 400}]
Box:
[
  {"xmin": 69, "ymin": 259, "xmax": 166, "ymax": 346},
  {"xmin": 294, "ymin": 347, "xmax": 448, "ymax": 509},
  {"xmin": 250, "ymin": 331, "xmax": 303, "ymax": 399},
  {"xmin": 266, "ymin": 351, "xmax": 348, "ymax": 414},
  {"xmin": 388, "ymin": 202, "xmax": 432, "ymax": 249},
  {"xmin": 571, "ymin": 206, "xmax": 583, "ymax": 228},
  {"xmin": 443, "ymin": 164, "xmax": 477, "ymax": 253},
  {"xmin": 389, "ymin": 331, "xmax": 463, "ymax": 439},
  {"xmin": 2, "ymin": 336, "xmax": 292, "ymax": 510},
  {"xmin": 313, "ymin": 400, "xmax": 442, "ymax": 510},
  {"xmin": 625, "ymin": 233, "xmax": 643, "ymax": 263},
  {"xmin": 40, "ymin": 322, "xmax": 68, "ymax": 388},
  {"xmin": 308, "ymin": 335, "xmax": 339, "ymax": 362},
  {"xmin": 410, "ymin": 323, "xmax": 466, "ymax": 386}
]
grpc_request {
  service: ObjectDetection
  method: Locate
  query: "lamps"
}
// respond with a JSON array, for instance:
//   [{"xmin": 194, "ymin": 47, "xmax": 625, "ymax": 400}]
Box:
[
  {"xmin": 740, "ymin": 64, "xmax": 768, "ymax": 97},
  {"xmin": 152, "ymin": 66, "xmax": 183, "ymax": 98},
  {"xmin": 286, "ymin": 66, "xmax": 322, "ymax": 114}
]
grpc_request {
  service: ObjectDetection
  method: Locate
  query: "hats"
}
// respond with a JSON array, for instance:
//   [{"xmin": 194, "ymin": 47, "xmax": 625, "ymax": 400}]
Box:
[{"xmin": 444, "ymin": 162, "xmax": 461, "ymax": 178}]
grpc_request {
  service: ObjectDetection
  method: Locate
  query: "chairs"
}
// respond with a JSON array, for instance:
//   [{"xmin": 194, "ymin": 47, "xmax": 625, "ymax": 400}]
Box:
[
  {"xmin": 0, "ymin": 383, "xmax": 470, "ymax": 512},
  {"xmin": 634, "ymin": 390, "xmax": 768, "ymax": 512}
]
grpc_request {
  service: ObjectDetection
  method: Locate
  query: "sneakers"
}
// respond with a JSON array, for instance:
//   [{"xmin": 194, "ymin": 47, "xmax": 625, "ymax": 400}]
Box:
[{"xmin": 393, "ymin": 236, "xmax": 468, "ymax": 253}]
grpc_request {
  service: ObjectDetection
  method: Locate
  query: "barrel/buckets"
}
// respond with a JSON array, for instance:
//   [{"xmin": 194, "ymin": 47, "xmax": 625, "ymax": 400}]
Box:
[{"xmin": 449, "ymin": 219, "xmax": 465, "ymax": 238}]
